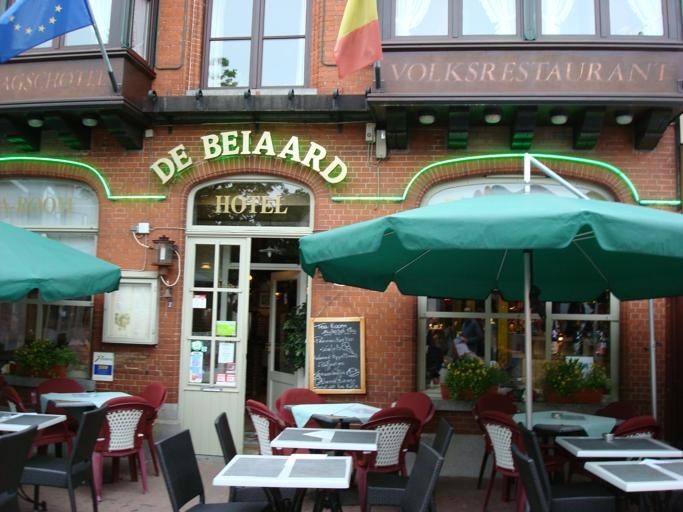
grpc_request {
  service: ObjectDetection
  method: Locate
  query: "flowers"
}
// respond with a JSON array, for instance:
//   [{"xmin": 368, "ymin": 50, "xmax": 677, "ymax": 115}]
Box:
[
  {"xmin": 445, "ymin": 355, "xmax": 509, "ymax": 402},
  {"xmin": 543, "ymin": 356, "xmax": 612, "ymax": 395},
  {"xmin": 12, "ymin": 339, "xmax": 79, "ymax": 378}
]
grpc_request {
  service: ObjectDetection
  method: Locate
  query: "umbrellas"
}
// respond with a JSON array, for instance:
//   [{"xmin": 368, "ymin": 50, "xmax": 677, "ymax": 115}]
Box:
[
  {"xmin": 1, "ymin": 221, "xmax": 121, "ymax": 303},
  {"xmin": 297, "ymin": 191, "xmax": 683, "ymax": 429}
]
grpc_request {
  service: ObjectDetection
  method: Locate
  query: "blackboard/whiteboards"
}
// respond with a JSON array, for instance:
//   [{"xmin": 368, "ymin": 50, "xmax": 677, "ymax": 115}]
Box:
[{"xmin": 308, "ymin": 316, "xmax": 366, "ymax": 395}]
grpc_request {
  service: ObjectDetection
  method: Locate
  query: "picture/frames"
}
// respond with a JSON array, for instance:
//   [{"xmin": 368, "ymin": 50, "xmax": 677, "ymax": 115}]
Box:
[{"xmin": 258, "ymin": 291, "xmax": 270, "ymax": 309}]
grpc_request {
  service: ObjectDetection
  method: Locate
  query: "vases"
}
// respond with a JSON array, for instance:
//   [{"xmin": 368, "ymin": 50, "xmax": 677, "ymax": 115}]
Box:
[{"xmin": 11, "ymin": 361, "xmax": 69, "ymax": 381}]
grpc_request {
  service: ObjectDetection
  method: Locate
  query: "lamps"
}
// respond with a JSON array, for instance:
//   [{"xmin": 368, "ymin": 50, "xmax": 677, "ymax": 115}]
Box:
[
  {"xmin": 551, "ymin": 111, "xmax": 568, "ymax": 126},
  {"xmin": 258, "ymin": 240, "xmax": 280, "ymax": 258},
  {"xmin": 418, "ymin": 111, "xmax": 435, "ymax": 125},
  {"xmin": 152, "ymin": 235, "xmax": 176, "ymax": 275},
  {"xmin": 27, "ymin": 117, "xmax": 44, "ymax": 127},
  {"xmin": 616, "ymin": 113, "xmax": 633, "ymax": 125},
  {"xmin": 147, "ymin": 88, "xmax": 371, "ymax": 101},
  {"xmin": 483, "ymin": 110, "xmax": 502, "ymax": 124},
  {"xmin": 82, "ymin": 117, "xmax": 98, "ymax": 127}
]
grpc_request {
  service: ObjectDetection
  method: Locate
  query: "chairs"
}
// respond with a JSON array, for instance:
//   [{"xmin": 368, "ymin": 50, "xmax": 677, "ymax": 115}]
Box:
[
  {"xmin": 154, "ymin": 389, "xmax": 454, "ymax": 512},
  {"xmin": 475, "ymin": 390, "xmax": 661, "ymax": 511},
  {"xmin": 0, "ymin": 375, "xmax": 166, "ymax": 512}
]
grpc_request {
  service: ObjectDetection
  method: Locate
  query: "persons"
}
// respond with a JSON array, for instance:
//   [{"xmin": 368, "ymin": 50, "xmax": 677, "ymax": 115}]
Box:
[{"xmin": 453, "ymin": 306, "xmax": 484, "ymax": 356}]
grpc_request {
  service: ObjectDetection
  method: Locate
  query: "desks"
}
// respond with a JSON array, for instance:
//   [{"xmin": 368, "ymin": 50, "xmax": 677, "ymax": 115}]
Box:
[
  {"xmin": 554, "ymin": 436, "xmax": 683, "ymax": 512},
  {"xmin": 249, "ymin": 344, "xmax": 268, "ymax": 398},
  {"xmin": 583, "ymin": 459, "xmax": 683, "ymax": 512}
]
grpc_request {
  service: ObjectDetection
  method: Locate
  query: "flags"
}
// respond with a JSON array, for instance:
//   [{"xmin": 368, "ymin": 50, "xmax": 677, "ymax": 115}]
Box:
[
  {"xmin": 1, "ymin": 0, "xmax": 92, "ymax": 60},
  {"xmin": 333, "ymin": 0, "xmax": 385, "ymax": 79}
]
